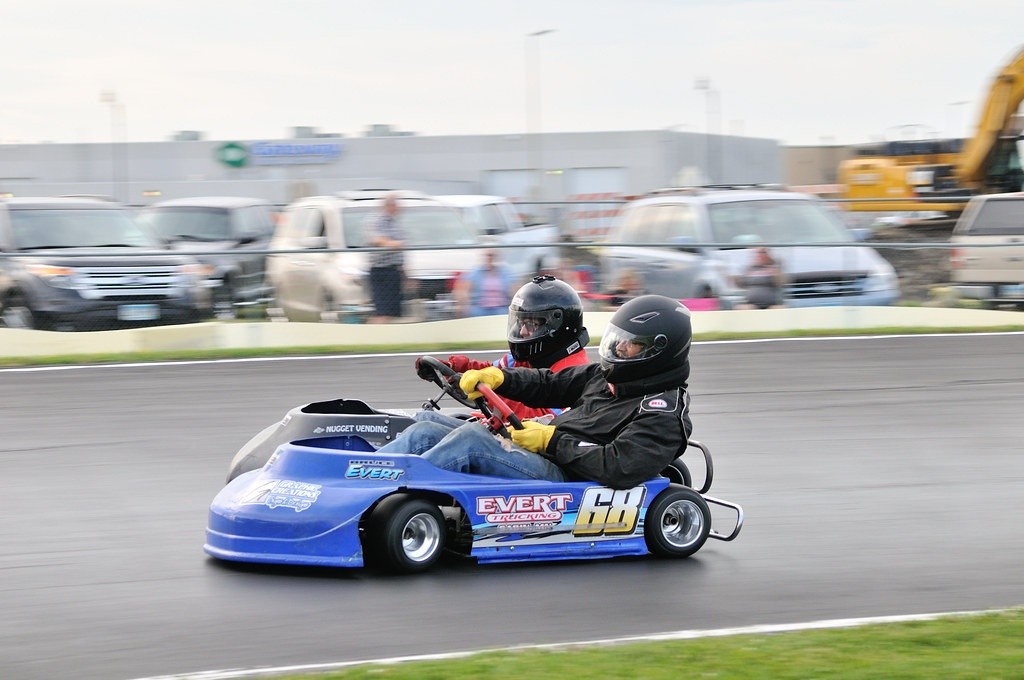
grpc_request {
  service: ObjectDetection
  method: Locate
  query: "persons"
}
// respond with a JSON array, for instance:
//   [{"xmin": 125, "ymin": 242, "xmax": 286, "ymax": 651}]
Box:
[
  {"xmin": 733, "ymin": 247, "xmax": 788, "ymax": 309},
  {"xmin": 610, "ymin": 266, "xmax": 651, "ymax": 307},
  {"xmin": 366, "ymin": 194, "xmax": 409, "ymax": 322},
  {"xmin": 450, "ymin": 247, "xmax": 571, "ymax": 320},
  {"xmin": 372, "ymin": 295, "xmax": 693, "ymax": 490},
  {"xmin": 414, "ymin": 274, "xmax": 590, "ymax": 430}
]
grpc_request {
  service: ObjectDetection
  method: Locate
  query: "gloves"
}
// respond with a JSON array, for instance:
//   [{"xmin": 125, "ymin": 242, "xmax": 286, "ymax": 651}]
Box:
[
  {"xmin": 508, "ymin": 421, "xmax": 556, "ymax": 453},
  {"xmin": 417, "ymin": 364, "xmax": 435, "ymax": 382},
  {"xmin": 448, "ymin": 372, "xmax": 468, "ymax": 400},
  {"xmin": 460, "ymin": 365, "xmax": 504, "ymax": 399}
]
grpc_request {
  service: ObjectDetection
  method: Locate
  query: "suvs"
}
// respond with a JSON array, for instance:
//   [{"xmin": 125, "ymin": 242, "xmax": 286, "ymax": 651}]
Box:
[
  {"xmin": 593, "ymin": 181, "xmax": 901, "ymax": 316},
  {"xmin": 139, "ymin": 194, "xmax": 276, "ymax": 326},
  {"xmin": 0, "ymin": 193, "xmax": 212, "ymax": 331},
  {"xmin": 940, "ymin": 192, "xmax": 1024, "ymax": 310}
]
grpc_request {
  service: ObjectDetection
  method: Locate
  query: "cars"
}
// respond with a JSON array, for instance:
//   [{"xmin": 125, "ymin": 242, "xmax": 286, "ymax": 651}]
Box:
[{"xmin": 263, "ymin": 189, "xmax": 573, "ymax": 326}]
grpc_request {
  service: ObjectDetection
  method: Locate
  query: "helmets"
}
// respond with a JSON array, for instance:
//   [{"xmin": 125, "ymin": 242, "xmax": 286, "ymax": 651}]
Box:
[
  {"xmin": 508, "ymin": 274, "xmax": 583, "ymax": 362},
  {"xmin": 598, "ymin": 294, "xmax": 691, "ymax": 384}
]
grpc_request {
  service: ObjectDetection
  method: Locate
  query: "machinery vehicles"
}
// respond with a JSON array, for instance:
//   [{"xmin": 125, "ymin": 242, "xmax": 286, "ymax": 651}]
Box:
[{"xmin": 830, "ymin": 37, "xmax": 1024, "ymax": 226}]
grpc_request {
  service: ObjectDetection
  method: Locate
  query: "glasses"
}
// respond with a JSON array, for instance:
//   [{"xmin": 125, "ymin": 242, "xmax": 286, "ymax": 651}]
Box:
[{"xmin": 517, "ymin": 319, "xmax": 545, "ymax": 332}]
